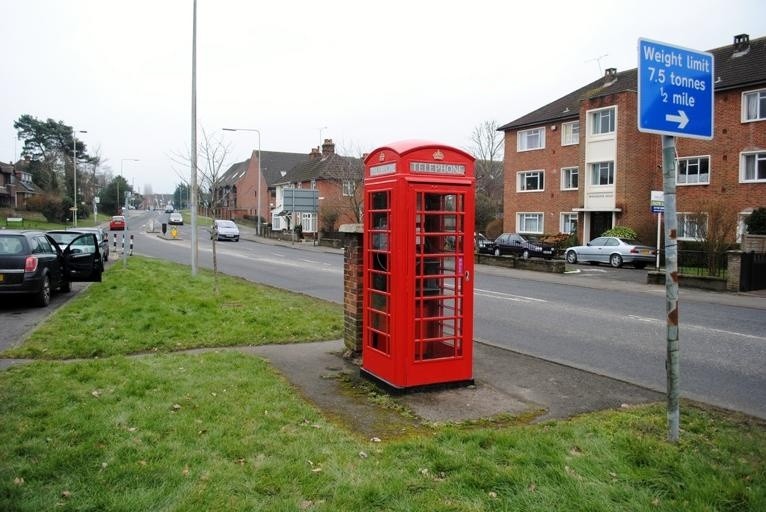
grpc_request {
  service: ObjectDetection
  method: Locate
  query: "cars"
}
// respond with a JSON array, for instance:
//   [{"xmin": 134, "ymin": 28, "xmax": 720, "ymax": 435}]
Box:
[
  {"xmin": 564, "ymin": 236, "xmax": 657, "ymax": 268},
  {"xmin": 211, "ymin": 219, "xmax": 240, "ymax": 242},
  {"xmin": 168, "ymin": 212, "xmax": 183, "ymax": 225},
  {"xmin": 110, "ymin": 215, "xmax": 125, "ymax": 230},
  {"xmin": 165, "ymin": 204, "xmax": 174, "ymax": 213},
  {"xmin": 0, "ymin": 228, "xmax": 110, "ymax": 307},
  {"xmin": 474, "ymin": 233, "xmax": 559, "ymax": 260}
]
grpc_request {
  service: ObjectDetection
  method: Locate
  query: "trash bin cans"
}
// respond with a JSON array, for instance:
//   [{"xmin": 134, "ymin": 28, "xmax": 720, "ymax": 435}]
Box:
[{"xmin": 162, "ymin": 224, "xmax": 167, "ymax": 235}]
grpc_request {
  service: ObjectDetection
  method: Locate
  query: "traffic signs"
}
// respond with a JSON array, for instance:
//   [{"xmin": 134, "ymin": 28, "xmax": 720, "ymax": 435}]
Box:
[{"xmin": 637, "ymin": 37, "xmax": 714, "ymax": 141}]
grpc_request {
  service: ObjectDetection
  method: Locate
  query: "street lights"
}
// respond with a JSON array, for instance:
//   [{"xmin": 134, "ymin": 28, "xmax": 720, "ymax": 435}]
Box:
[
  {"xmin": 120, "ymin": 159, "xmax": 140, "ymax": 187},
  {"xmin": 73, "ymin": 129, "xmax": 88, "ymax": 228},
  {"xmin": 222, "ymin": 128, "xmax": 261, "ymax": 235}
]
